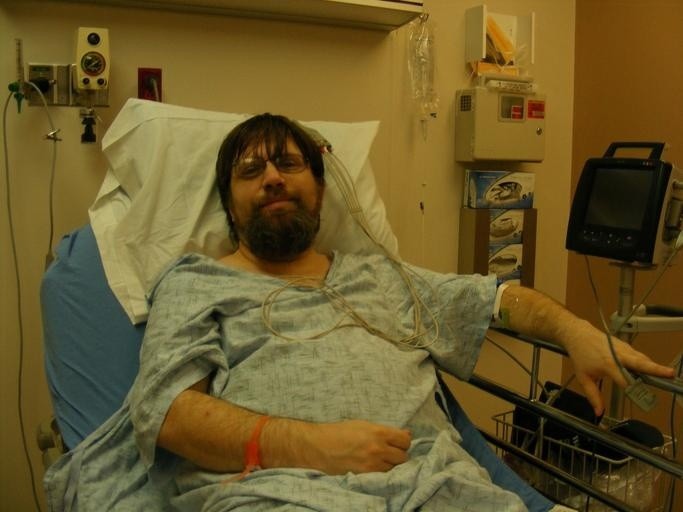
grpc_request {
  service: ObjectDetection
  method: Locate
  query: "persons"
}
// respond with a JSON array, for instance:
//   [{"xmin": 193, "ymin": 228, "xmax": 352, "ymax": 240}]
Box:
[{"xmin": 129, "ymin": 114, "xmax": 675, "ymax": 512}]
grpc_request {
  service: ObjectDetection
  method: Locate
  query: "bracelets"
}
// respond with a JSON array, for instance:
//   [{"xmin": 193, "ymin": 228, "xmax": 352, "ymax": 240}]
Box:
[{"xmin": 245, "ymin": 415, "xmax": 273, "ymax": 473}]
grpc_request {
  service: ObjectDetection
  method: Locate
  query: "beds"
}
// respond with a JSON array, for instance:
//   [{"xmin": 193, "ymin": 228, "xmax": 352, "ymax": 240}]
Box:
[{"xmin": 37, "ymin": 97, "xmax": 586, "ymax": 512}]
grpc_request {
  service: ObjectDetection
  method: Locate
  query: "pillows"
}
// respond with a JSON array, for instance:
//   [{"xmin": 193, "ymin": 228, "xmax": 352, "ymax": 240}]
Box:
[{"xmin": 88, "ymin": 96, "xmax": 400, "ymax": 328}]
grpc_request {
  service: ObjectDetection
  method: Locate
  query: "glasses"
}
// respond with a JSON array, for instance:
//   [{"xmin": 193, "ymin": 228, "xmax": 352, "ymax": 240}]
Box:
[{"xmin": 226, "ymin": 151, "xmax": 309, "ymax": 182}]
